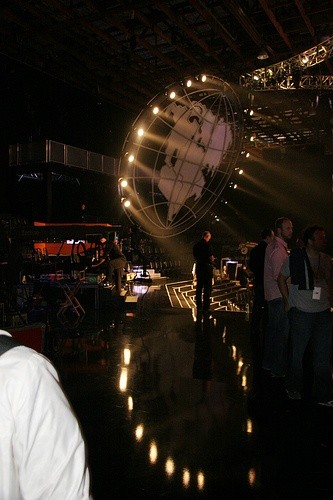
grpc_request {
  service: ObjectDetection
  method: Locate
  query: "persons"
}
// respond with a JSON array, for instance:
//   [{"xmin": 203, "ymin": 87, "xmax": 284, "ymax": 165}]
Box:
[
  {"xmin": 0, "ymin": 327, "xmax": 95, "ymax": 500},
  {"xmin": 193, "ymin": 231, "xmax": 217, "ymax": 313},
  {"xmin": 92, "ymin": 237, "xmax": 127, "ymax": 293},
  {"xmin": 240, "ymin": 217, "xmax": 333, "ymax": 407}
]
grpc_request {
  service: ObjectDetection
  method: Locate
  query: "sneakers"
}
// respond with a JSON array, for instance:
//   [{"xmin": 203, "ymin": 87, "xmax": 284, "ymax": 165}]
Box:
[
  {"xmin": 318, "ymin": 397, "xmax": 332, "ymax": 408},
  {"xmin": 285, "ymin": 388, "xmax": 300, "ymax": 401},
  {"xmin": 263, "ymin": 363, "xmax": 287, "ymax": 379}
]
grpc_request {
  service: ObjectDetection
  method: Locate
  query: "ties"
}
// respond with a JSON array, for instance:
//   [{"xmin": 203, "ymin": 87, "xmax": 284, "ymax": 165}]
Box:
[{"xmin": 281, "ymin": 243, "xmax": 289, "ymax": 256}]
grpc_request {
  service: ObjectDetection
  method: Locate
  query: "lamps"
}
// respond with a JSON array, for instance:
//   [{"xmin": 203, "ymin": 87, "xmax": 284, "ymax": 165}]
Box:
[
  {"xmin": 121, "ymin": 150, "xmax": 135, "ymax": 167},
  {"xmin": 165, "ymin": 91, "xmax": 177, "ymax": 100},
  {"xmin": 183, "ymin": 78, "xmax": 193, "ymax": 88},
  {"xmin": 117, "ymin": 176, "xmax": 128, "ymax": 190},
  {"xmin": 195, "ymin": 72, "xmax": 207, "ymax": 83},
  {"xmin": 149, "ymin": 105, "xmax": 161, "ymax": 115},
  {"xmin": 119, "ymin": 196, "xmax": 131, "ymax": 208},
  {"xmin": 257, "ymin": 48, "xmax": 270, "ymax": 62}
]
